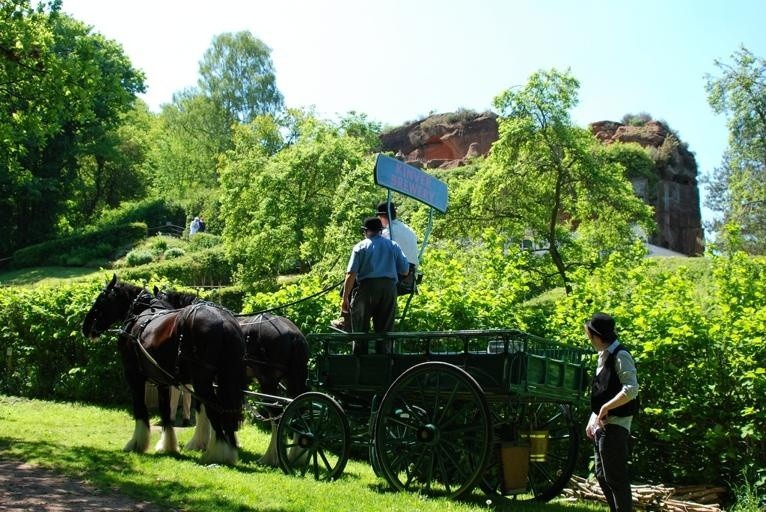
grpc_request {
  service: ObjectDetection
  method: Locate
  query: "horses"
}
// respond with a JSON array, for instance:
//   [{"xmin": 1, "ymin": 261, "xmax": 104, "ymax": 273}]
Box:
[
  {"xmin": 152, "ymin": 284, "xmax": 310, "ymax": 470},
  {"xmin": 81, "ymin": 272, "xmax": 247, "ymax": 467}
]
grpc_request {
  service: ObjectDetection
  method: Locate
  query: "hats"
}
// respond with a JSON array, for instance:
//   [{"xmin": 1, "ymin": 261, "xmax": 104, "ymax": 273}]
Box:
[
  {"xmin": 585, "ymin": 312, "xmax": 618, "ymax": 340},
  {"xmin": 375, "ymin": 202, "xmax": 395, "ymax": 214},
  {"xmin": 359, "ymin": 217, "xmax": 384, "ymax": 230}
]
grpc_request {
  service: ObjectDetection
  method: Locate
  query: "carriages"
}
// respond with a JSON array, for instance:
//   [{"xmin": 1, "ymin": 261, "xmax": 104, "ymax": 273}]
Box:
[{"xmin": 81, "ymin": 150, "xmax": 596, "ymax": 509}]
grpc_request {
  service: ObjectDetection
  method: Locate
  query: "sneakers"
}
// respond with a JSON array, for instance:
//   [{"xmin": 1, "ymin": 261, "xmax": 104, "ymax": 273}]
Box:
[{"xmin": 330, "ymin": 313, "xmax": 352, "ymax": 333}]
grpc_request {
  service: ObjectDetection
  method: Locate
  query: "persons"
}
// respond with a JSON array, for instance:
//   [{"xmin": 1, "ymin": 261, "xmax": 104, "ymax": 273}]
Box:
[
  {"xmin": 583, "ymin": 310, "xmax": 642, "ymax": 512},
  {"xmin": 339, "ymin": 216, "xmax": 411, "ymax": 357},
  {"xmin": 329, "ymin": 200, "xmax": 421, "ymax": 335},
  {"xmin": 198, "ymin": 215, "xmax": 206, "ymax": 232},
  {"xmin": 189, "ymin": 215, "xmax": 199, "ymax": 237}
]
